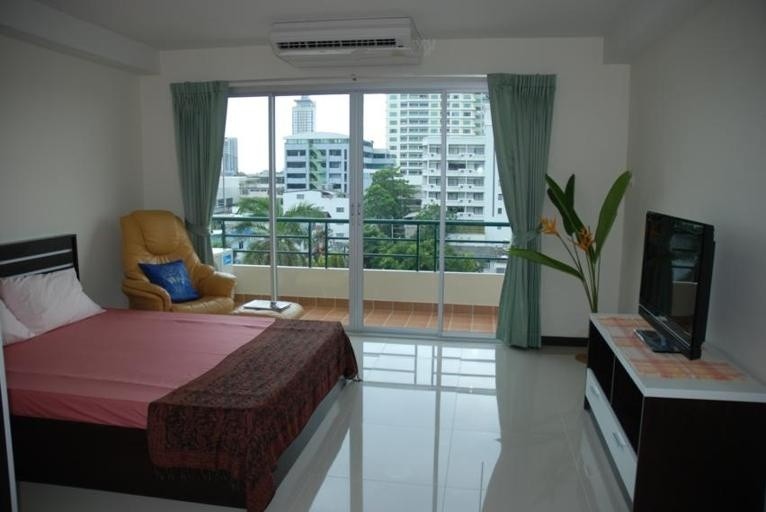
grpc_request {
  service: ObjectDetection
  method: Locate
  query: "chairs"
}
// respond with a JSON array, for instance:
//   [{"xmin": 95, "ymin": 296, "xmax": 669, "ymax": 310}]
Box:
[{"xmin": 118, "ymin": 208, "xmax": 236, "ymax": 315}]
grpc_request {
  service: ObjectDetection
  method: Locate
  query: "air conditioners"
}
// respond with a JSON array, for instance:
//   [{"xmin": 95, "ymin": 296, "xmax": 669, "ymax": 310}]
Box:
[{"xmin": 269, "ymin": 17, "xmax": 422, "ymax": 71}]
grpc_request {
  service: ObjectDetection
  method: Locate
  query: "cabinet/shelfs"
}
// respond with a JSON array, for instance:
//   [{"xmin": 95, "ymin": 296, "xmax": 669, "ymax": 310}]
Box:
[{"xmin": 582, "ymin": 315, "xmax": 765, "ymax": 511}]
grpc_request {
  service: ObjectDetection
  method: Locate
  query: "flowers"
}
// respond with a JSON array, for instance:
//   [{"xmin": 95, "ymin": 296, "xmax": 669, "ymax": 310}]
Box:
[{"xmin": 503, "ymin": 168, "xmax": 656, "ymax": 312}]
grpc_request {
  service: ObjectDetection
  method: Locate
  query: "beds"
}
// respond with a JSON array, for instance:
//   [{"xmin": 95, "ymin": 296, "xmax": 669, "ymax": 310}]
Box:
[{"xmin": 0, "ymin": 232, "xmax": 361, "ymax": 512}]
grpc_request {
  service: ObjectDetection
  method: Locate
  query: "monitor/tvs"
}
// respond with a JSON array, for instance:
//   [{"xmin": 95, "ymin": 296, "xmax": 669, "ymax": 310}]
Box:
[{"xmin": 634, "ymin": 209, "xmax": 716, "ymax": 361}]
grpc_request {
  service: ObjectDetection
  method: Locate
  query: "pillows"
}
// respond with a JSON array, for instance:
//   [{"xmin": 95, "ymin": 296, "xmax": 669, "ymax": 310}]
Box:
[
  {"xmin": 136, "ymin": 257, "xmax": 201, "ymax": 302},
  {"xmin": 0, "ymin": 268, "xmax": 108, "ymax": 348}
]
